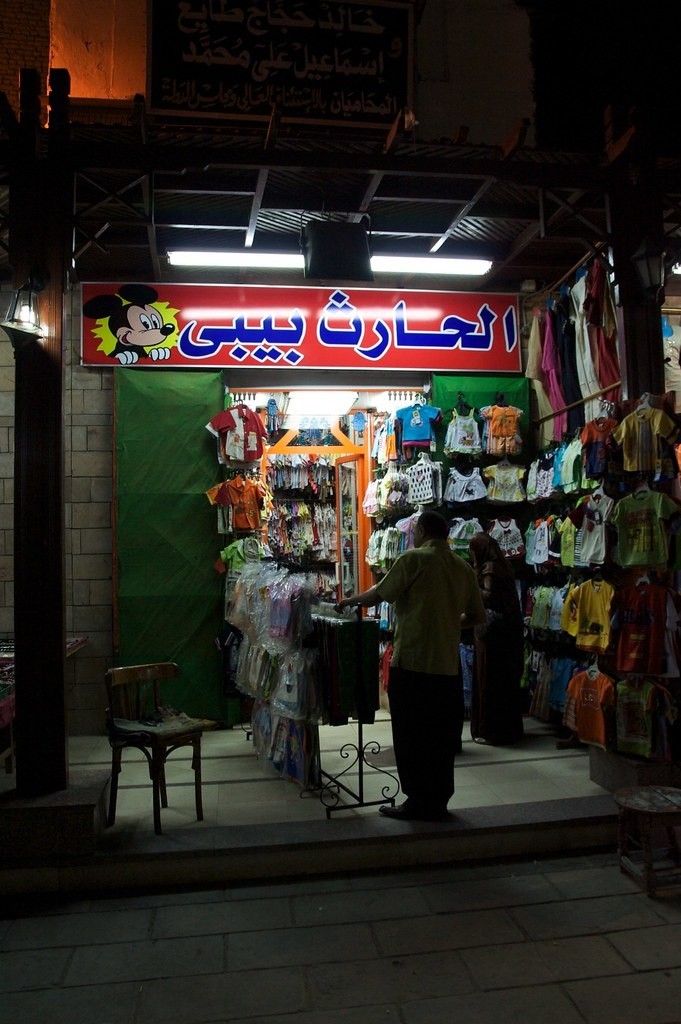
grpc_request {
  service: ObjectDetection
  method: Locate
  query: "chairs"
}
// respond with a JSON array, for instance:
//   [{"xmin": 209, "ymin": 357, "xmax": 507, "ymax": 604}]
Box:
[{"xmin": 105, "ymin": 662, "xmax": 216, "ymax": 835}]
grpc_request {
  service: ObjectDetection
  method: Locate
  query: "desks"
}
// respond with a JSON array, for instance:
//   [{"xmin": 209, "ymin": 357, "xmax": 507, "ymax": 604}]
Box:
[{"xmin": 614, "ymin": 785, "xmax": 681, "ymax": 899}]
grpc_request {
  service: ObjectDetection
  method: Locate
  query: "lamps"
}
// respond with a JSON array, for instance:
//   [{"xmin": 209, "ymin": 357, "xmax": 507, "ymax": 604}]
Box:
[{"xmin": 300, "ymin": 203, "xmax": 377, "ymax": 283}]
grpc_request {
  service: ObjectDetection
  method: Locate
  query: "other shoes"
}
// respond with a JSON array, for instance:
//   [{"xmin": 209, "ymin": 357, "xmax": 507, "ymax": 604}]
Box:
[{"xmin": 475, "ymin": 737, "xmax": 487, "ymax": 743}]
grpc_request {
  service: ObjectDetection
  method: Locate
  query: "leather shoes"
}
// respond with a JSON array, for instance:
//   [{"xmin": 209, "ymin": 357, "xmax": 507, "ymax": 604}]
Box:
[{"xmin": 379, "ymin": 799, "xmax": 419, "ymax": 819}]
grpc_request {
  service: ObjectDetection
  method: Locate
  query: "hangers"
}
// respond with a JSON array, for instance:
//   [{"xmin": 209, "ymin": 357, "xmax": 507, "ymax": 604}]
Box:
[{"xmin": 229, "ymin": 394, "xmax": 669, "ymax": 692}]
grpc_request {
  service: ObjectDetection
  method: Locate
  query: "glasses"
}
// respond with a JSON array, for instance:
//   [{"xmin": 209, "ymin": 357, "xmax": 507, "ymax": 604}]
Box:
[{"xmin": 410, "ymin": 527, "xmax": 422, "ymax": 535}]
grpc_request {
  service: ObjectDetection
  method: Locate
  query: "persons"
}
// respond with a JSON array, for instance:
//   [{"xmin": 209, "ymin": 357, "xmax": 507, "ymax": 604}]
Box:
[
  {"xmin": 466, "ymin": 531, "xmax": 526, "ymax": 746},
  {"xmin": 333, "ymin": 509, "xmax": 489, "ymax": 821}
]
